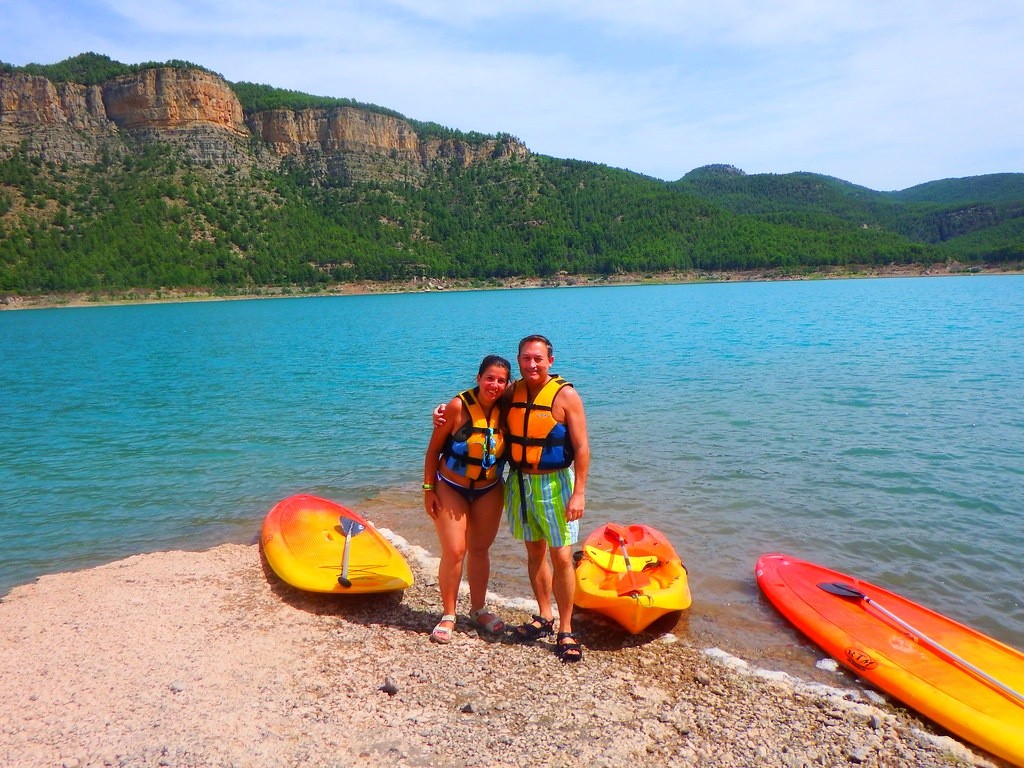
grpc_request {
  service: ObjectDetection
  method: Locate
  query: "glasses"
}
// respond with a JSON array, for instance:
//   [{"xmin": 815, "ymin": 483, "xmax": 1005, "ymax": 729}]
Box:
[{"xmin": 482, "ymin": 428, "xmax": 496, "ymax": 469}]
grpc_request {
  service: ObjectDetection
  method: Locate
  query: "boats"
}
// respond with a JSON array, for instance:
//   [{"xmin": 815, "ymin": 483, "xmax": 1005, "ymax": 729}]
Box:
[{"xmin": 568, "ymin": 522, "xmax": 693, "ymax": 636}]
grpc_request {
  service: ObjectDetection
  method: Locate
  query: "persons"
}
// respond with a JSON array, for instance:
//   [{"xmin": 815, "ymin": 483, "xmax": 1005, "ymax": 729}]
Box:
[
  {"xmin": 433, "ymin": 334, "xmax": 589, "ymax": 661},
  {"xmin": 423, "ymin": 355, "xmax": 510, "ymax": 645}
]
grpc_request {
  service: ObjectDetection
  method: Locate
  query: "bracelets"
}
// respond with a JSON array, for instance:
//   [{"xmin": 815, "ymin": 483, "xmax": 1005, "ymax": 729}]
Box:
[{"xmin": 423, "ymin": 484, "xmax": 433, "ymax": 491}]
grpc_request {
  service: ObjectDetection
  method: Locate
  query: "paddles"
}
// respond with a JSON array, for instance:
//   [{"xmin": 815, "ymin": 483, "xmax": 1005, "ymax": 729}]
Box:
[
  {"xmin": 607, "ymin": 523, "xmax": 651, "ymax": 596},
  {"xmin": 336, "ymin": 513, "xmax": 368, "ymax": 587},
  {"xmin": 817, "ymin": 581, "xmax": 1024, "ymax": 706}
]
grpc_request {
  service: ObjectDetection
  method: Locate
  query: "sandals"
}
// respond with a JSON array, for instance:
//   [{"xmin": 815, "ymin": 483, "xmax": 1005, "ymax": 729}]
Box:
[
  {"xmin": 557, "ymin": 631, "xmax": 582, "ymax": 662},
  {"xmin": 432, "ymin": 611, "xmax": 456, "ymax": 643},
  {"xmin": 516, "ymin": 614, "xmax": 555, "ymax": 640},
  {"xmin": 469, "ymin": 607, "xmax": 506, "ymax": 635}
]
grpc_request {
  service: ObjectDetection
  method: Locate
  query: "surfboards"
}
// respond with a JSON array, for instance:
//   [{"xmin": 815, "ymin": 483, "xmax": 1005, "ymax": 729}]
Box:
[
  {"xmin": 261, "ymin": 494, "xmax": 414, "ymax": 594},
  {"xmin": 754, "ymin": 554, "xmax": 1024, "ymax": 768}
]
